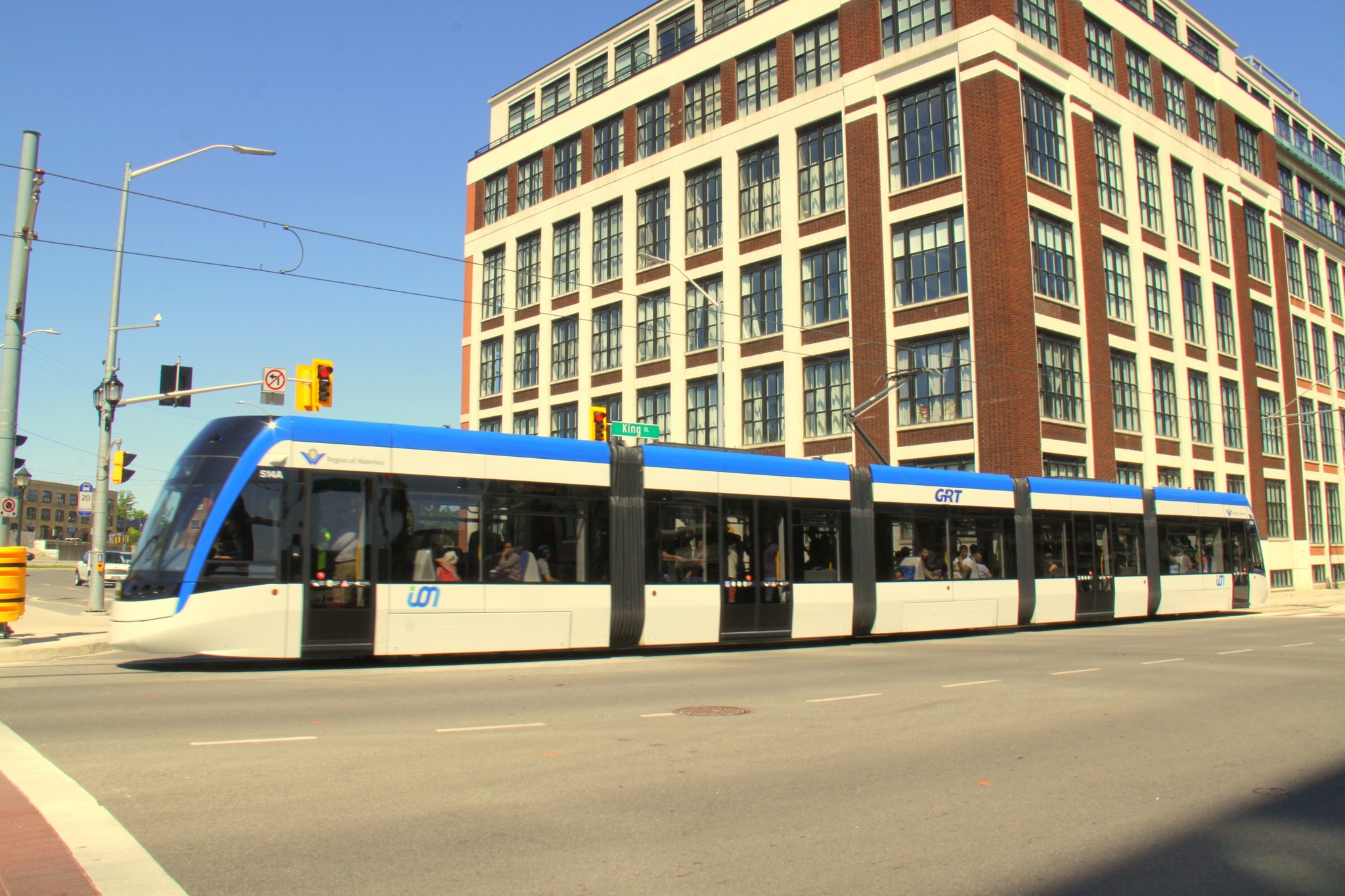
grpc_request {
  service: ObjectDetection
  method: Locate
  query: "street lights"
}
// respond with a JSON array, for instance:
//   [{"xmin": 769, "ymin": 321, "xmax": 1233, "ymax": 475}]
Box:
[
  {"xmin": 122, "ymin": 502, "xmax": 137, "ymax": 551},
  {"xmin": 82, "ymin": 143, "xmax": 277, "ymax": 619},
  {"xmin": 13, "ymin": 464, "xmax": 32, "ymax": 548}
]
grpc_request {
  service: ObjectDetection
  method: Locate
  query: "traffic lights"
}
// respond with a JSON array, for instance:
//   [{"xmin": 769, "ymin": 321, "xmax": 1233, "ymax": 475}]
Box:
[
  {"xmin": 15, "ymin": 432, "xmax": 28, "ymax": 471},
  {"xmin": 591, "ymin": 406, "xmax": 610, "ymax": 442},
  {"xmin": 309, "ymin": 358, "xmax": 334, "ymax": 409},
  {"xmin": 110, "ymin": 449, "xmax": 139, "ymax": 487}
]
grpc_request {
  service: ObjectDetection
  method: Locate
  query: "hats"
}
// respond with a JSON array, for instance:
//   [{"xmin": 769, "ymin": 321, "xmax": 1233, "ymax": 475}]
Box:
[{"xmin": 443, "ymin": 551, "xmax": 459, "ymax": 565}]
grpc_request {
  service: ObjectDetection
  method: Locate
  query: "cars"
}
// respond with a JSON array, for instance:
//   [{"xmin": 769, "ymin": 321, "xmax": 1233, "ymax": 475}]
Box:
[
  {"xmin": 75, "ymin": 550, "xmax": 135, "ymax": 587},
  {"xmin": 64, "ymin": 538, "xmax": 81, "ymax": 542},
  {"xmin": 122, "ymin": 552, "xmax": 135, "ymax": 565}
]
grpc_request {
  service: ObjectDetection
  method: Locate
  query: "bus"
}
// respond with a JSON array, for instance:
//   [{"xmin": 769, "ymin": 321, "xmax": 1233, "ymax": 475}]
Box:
[{"xmin": 112, "ymin": 415, "xmax": 1268, "ymax": 661}]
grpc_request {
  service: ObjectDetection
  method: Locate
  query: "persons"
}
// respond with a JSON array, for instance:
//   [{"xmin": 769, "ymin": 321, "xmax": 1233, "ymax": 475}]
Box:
[
  {"xmin": 658, "ymin": 519, "xmax": 831, "ymax": 604},
  {"xmin": 893, "ymin": 528, "xmax": 1243, "ymax": 580},
  {"xmin": 331, "ymin": 523, "xmax": 358, "ymax": 603},
  {"xmin": 223, "ymin": 518, "xmax": 255, "ymax": 562},
  {"xmin": 434, "ymin": 509, "xmax": 564, "ymax": 585}
]
